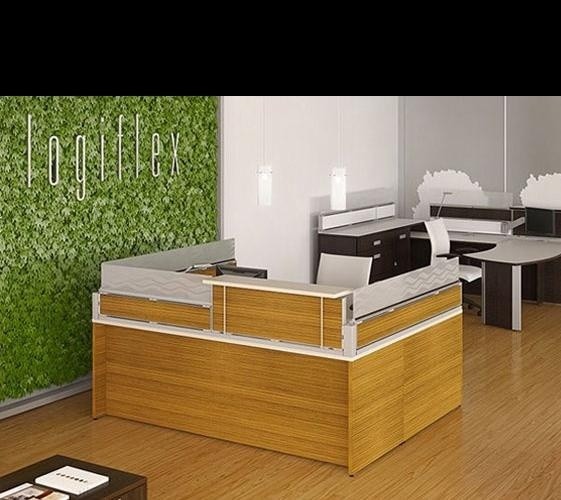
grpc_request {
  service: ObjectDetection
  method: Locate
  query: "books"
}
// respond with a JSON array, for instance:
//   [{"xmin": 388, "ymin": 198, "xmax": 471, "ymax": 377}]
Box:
[
  {"xmin": 35, "ymin": 465, "xmax": 109, "ymax": 495},
  {"xmin": 0, "ymin": 483, "xmax": 69, "ymax": 500}
]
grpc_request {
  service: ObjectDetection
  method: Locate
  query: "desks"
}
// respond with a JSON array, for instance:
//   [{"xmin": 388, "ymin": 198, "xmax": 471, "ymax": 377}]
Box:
[
  {"xmin": 399, "ymin": 232, "xmax": 560, "ymax": 332},
  {"xmin": 0, "ymin": 453, "xmax": 152, "ymax": 499}
]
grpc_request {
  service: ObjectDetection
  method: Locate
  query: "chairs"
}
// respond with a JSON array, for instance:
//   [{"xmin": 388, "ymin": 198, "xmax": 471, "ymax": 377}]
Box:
[
  {"xmin": 315, "ymin": 252, "xmax": 374, "ymax": 291},
  {"xmin": 422, "ymin": 217, "xmax": 483, "ymax": 317}
]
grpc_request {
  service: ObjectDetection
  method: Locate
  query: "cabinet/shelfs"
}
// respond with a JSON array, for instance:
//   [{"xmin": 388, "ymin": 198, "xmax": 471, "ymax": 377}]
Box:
[{"xmin": 318, "ymin": 217, "xmax": 426, "ymax": 285}]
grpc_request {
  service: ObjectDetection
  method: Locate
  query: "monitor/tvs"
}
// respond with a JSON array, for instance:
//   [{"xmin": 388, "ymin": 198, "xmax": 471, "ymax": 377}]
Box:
[{"xmin": 215, "ymin": 265, "xmax": 267, "ymax": 279}]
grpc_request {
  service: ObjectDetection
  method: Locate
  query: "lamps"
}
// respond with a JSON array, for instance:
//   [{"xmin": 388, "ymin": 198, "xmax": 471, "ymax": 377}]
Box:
[
  {"xmin": 256, "ymin": 96, "xmax": 273, "ymax": 209},
  {"xmin": 331, "ymin": 96, "xmax": 349, "ymax": 213}
]
